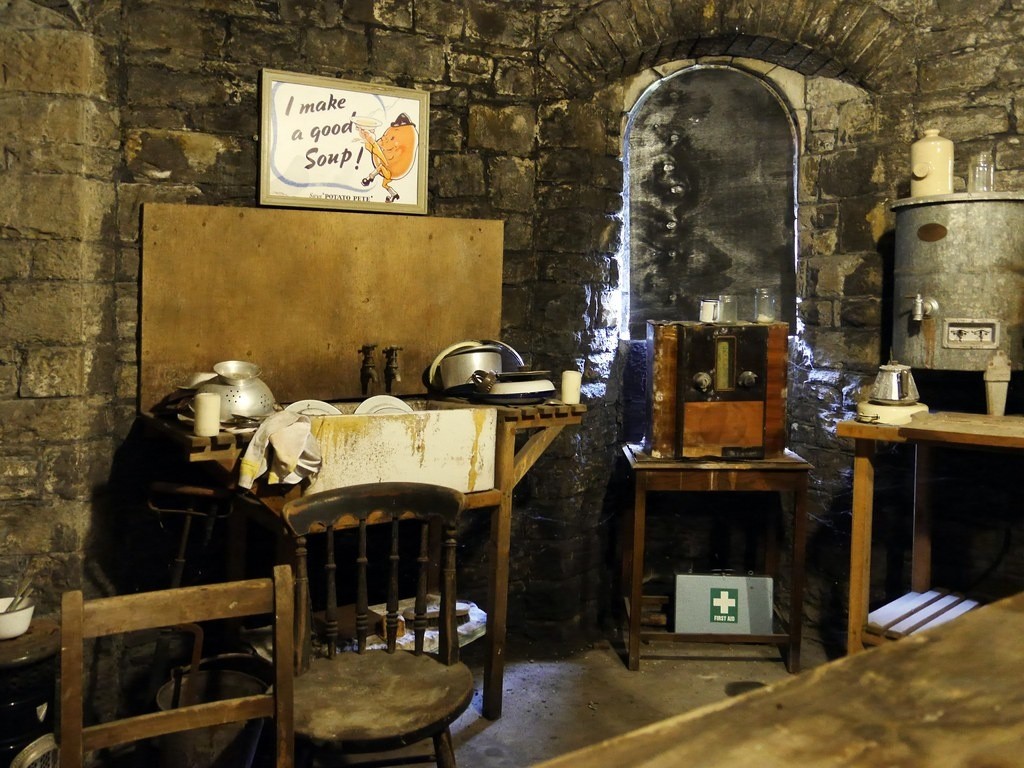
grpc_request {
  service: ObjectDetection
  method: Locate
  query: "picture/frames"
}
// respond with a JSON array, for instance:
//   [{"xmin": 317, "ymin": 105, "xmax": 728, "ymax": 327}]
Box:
[{"xmin": 260, "ymin": 68, "xmax": 430, "ymax": 214}]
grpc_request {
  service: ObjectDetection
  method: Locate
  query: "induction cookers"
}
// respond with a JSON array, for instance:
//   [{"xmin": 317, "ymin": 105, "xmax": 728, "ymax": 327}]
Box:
[{"xmin": 856, "ymin": 399, "xmax": 929, "ymax": 425}]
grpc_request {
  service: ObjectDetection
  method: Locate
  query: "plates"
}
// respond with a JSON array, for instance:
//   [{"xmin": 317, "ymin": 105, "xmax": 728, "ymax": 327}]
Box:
[
  {"xmin": 480, "ymin": 339, "xmax": 524, "ymax": 372},
  {"xmin": 284, "ymin": 399, "xmax": 343, "ymax": 415},
  {"xmin": 353, "ymin": 394, "xmax": 413, "ymax": 415},
  {"xmin": 439, "ymin": 352, "xmax": 503, "ymax": 397},
  {"xmin": 471, "ymin": 389, "xmax": 550, "ymax": 405},
  {"xmin": 429, "ymin": 340, "xmax": 482, "ymax": 389},
  {"xmin": 489, "ymin": 379, "xmax": 556, "ymax": 395}
]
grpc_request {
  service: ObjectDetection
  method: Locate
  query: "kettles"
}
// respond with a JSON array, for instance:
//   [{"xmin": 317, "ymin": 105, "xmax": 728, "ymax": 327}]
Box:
[{"xmin": 867, "ymin": 359, "xmax": 920, "ymax": 406}]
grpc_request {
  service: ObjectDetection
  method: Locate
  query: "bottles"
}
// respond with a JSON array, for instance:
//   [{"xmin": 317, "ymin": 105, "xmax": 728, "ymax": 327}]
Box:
[
  {"xmin": 754, "ymin": 287, "xmax": 776, "ymax": 325},
  {"xmin": 717, "ymin": 294, "xmax": 737, "ymax": 326}
]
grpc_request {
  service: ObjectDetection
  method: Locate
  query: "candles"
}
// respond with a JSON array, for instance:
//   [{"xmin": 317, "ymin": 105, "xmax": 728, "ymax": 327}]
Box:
[
  {"xmin": 195, "ymin": 393, "xmax": 221, "ymax": 436},
  {"xmin": 562, "ymin": 371, "xmax": 582, "ymax": 404}
]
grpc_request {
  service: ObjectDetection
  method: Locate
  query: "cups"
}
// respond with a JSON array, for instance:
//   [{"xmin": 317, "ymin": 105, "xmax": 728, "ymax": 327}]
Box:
[{"xmin": 698, "ymin": 299, "xmax": 725, "ymax": 323}]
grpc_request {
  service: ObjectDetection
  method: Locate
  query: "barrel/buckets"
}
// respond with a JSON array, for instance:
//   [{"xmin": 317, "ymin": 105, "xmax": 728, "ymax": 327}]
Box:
[{"xmin": 155, "ymin": 652, "xmax": 273, "ymax": 768}]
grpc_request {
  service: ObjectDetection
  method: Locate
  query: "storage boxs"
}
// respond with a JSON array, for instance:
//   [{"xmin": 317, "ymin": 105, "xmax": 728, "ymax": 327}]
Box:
[
  {"xmin": 664, "ymin": 556, "xmax": 774, "ymax": 634},
  {"xmin": 278, "ymin": 401, "xmax": 497, "ymax": 498}
]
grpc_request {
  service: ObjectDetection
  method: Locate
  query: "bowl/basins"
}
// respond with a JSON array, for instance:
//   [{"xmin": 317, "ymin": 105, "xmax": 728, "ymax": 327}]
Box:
[{"xmin": 0, "ymin": 597, "xmax": 35, "ymax": 640}]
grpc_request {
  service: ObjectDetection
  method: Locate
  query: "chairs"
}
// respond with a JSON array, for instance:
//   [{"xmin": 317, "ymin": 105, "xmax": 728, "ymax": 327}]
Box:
[
  {"xmin": 279, "ymin": 482, "xmax": 474, "ymax": 768},
  {"xmin": 60, "ymin": 565, "xmax": 295, "ymax": 768}
]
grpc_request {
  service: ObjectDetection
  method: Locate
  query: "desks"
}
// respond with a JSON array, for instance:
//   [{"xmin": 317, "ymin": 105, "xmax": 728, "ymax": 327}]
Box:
[
  {"xmin": 144, "ymin": 397, "xmax": 587, "ymax": 721},
  {"xmin": 836, "ymin": 411, "xmax": 1024, "ymax": 655},
  {"xmin": 529, "ymin": 591, "xmax": 1024, "ymax": 768},
  {"xmin": 620, "ymin": 444, "xmax": 816, "ymax": 674},
  {"xmin": 0, "ymin": 614, "xmax": 59, "ymax": 735}
]
